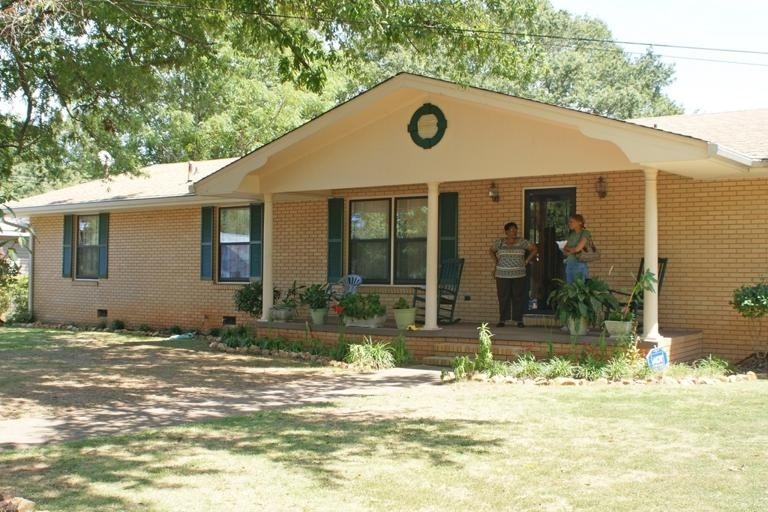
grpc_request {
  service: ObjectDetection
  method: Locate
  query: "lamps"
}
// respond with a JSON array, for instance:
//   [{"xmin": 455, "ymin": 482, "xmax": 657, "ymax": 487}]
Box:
[
  {"xmin": 488, "ymin": 181, "xmax": 500, "ymax": 203},
  {"xmin": 594, "ymin": 176, "xmax": 608, "ymax": 199}
]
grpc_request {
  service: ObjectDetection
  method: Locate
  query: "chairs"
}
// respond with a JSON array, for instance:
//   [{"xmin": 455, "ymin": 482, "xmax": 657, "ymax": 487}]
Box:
[
  {"xmin": 329, "ymin": 273, "xmax": 363, "ymax": 300},
  {"xmin": 414, "ymin": 255, "xmax": 467, "ymax": 326},
  {"xmin": 605, "ymin": 256, "xmax": 669, "ymax": 332}
]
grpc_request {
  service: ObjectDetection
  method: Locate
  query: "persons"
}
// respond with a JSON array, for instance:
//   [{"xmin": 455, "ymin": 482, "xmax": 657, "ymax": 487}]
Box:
[
  {"xmin": 490, "ymin": 222, "xmax": 538, "ymax": 328},
  {"xmin": 564, "ymin": 214, "xmax": 592, "ymax": 287}
]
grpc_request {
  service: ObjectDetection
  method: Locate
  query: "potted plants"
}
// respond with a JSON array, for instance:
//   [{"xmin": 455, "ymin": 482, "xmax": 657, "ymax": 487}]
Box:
[
  {"xmin": 337, "ymin": 292, "xmax": 387, "ymax": 329},
  {"xmin": 546, "ymin": 269, "xmax": 658, "ymax": 339},
  {"xmin": 392, "ymin": 297, "xmax": 418, "ymax": 329},
  {"xmin": 273, "ymin": 280, "xmax": 335, "ymax": 326}
]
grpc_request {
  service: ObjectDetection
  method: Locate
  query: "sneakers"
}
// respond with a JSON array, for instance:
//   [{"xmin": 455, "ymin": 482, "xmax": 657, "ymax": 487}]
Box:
[
  {"xmin": 496, "ymin": 322, "xmax": 504, "ymax": 326},
  {"xmin": 517, "ymin": 322, "xmax": 523, "ymax": 327}
]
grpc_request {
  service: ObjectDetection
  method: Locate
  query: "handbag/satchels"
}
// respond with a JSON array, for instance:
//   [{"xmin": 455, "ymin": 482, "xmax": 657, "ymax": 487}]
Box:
[{"xmin": 574, "ymin": 230, "xmax": 600, "ymax": 262}]
